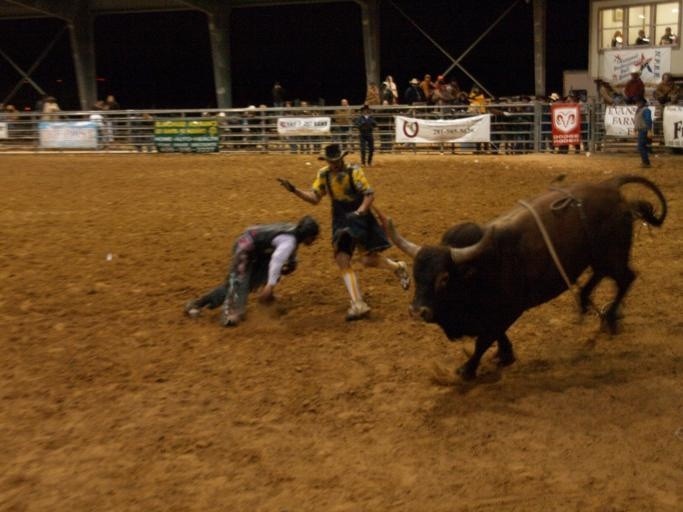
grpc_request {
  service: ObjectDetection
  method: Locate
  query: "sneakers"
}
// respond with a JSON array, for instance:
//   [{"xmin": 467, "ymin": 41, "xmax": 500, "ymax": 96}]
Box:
[
  {"xmin": 344, "ymin": 304, "xmax": 372, "ymax": 323},
  {"xmin": 395, "ymin": 260, "xmax": 410, "ymax": 290}
]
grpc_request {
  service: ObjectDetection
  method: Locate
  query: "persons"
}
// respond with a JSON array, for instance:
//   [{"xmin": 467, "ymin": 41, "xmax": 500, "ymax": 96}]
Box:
[
  {"xmin": 624, "ymin": 70, "xmax": 680, "ymax": 167},
  {"xmin": 92, "ymin": 96, "xmax": 120, "ymax": 143},
  {"xmin": 277, "ymin": 143, "xmax": 410, "ymax": 320},
  {"xmin": 201, "ymin": 104, "xmax": 272, "ymax": 150},
  {"xmin": 183, "ymin": 213, "xmax": 321, "ymax": 326},
  {"xmin": 381, "ymin": 75, "xmax": 622, "ymax": 155},
  {"xmin": 3, "ymin": 96, "xmax": 61, "ymax": 121},
  {"xmin": 131, "ymin": 114, "xmax": 156, "ymax": 153},
  {"xmin": 283, "ymin": 101, "xmax": 320, "ymax": 153},
  {"xmin": 333, "ymin": 82, "xmax": 381, "ymax": 166},
  {"xmin": 611, "ymin": 27, "xmax": 677, "ymax": 48}
]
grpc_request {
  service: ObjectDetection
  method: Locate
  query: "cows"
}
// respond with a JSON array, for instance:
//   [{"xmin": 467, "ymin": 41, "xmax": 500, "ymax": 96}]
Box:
[{"xmin": 382, "ymin": 172, "xmax": 668, "ymax": 382}]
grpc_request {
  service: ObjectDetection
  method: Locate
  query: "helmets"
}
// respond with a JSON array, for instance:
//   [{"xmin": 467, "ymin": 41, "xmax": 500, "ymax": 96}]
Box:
[{"xmin": 299, "ymin": 215, "xmax": 319, "ymax": 245}]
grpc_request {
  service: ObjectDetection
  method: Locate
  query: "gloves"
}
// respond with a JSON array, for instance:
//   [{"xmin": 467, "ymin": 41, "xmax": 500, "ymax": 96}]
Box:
[
  {"xmin": 344, "ymin": 210, "xmax": 359, "ymax": 220},
  {"xmin": 277, "ymin": 177, "xmax": 295, "ymax": 192}
]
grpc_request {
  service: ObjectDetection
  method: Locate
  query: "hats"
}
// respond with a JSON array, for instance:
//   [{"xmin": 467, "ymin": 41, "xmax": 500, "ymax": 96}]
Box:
[
  {"xmin": 629, "ymin": 67, "xmax": 642, "ymax": 76},
  {"xmin": 317, "ymin": 144, "xmax": 349, "ymax": 163},
  {"xmin": 409, "ymin": 78, "xmax": 418, "ymax": 85}
]
